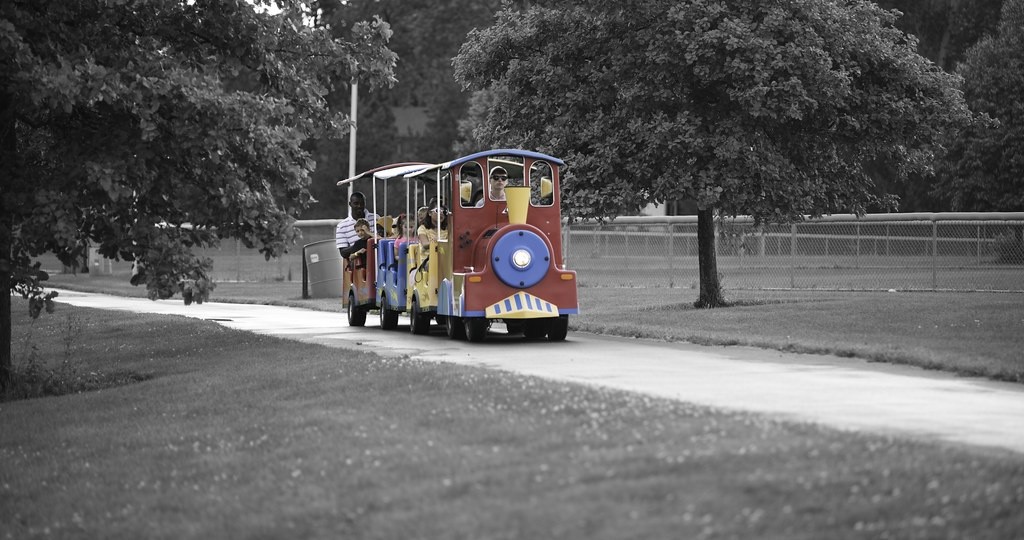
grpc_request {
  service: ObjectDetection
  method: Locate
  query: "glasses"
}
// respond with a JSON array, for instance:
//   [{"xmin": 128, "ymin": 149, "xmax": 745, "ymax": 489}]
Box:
[
  {"xmin": 490, "ymin": 175, "xmax": 507, "ymax": 181},
  {"xmin": 431, "ymin": 208, "xmax": 448, "ymax": 216}
]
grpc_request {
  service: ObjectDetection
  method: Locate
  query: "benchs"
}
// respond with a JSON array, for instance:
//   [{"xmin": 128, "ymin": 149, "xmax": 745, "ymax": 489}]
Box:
[
  {"xmin": 398, "ymin": 242, "xmax": 419, "ymax": 286},
  {"xmin": 430, "ymin": 243, "xmax": 448, "ymax": 288},
  {"xmin": 342, "ymin": 259, "xmax": 352, "ymax": 282},
  {"xmin": 353, "ymin": 259, "xmax": 366, "ymax": 280},
  {"xmin": 386, "ymin": 242, "xmax": 397, "ymax": 282},
  {"xmin": 408, "ymin": 243, "xmax": 428, "ymax": 286},
  {"xmin": 376, "ymin": 239, "xmax": 394, "ymax": 280}
]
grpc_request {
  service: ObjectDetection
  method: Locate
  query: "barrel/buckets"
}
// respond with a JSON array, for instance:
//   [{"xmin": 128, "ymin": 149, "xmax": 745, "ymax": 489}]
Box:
[{"xmin": 304, "ymin": 240, "xmax": 343, "ymax": 299}]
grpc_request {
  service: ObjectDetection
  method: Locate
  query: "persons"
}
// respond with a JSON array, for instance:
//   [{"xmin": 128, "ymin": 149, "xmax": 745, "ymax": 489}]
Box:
[
  {"xmin": 429, "ymin": 195, "xmax": 447, "ymax": 206},
  {"xmin": 476, "ymin": 166, "xmax": 509, "ymax": 207},
  {"xmin": 394, "ymin": 214, "xmax": 419, "ymax": 260},
  {"xmin": 348, "ymin": 218, "xmax": 381, "ymax": 267},
  {"xmin": 416, "ymin": 202, "xmax": 448, "ymax": 272},
  {"xmin": 418, "ymin": 207, "xmax": 429, "ymax": 225},
  {"xmin": 336, "ymin": 191, "xmax": 380, "ymax": 259},
  {"xmin": 461, "ymin": 167, "xmax": 483, "ymax": 206},
  {"xmin": 376, "ymin": 215, "xmax": 393, "ymax": 237}
]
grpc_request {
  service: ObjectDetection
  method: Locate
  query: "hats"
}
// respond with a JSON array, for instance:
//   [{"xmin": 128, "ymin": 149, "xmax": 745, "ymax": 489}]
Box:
[
  {"xmin": 489, "ymin": 165, "xmax": 508, "ymax": 176},
  {"xmin": 377, "ymin": 215, "xmax": 393, "ymax": 235}
]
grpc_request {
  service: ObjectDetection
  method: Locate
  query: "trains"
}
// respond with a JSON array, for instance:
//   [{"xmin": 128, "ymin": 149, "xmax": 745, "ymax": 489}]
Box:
[{"xmin": 336, "ymin": 148, "xmax": 579, "ymax": 344}]
